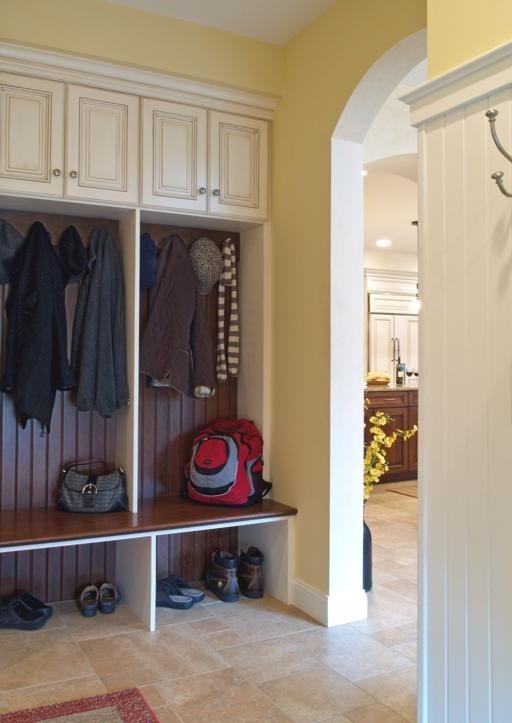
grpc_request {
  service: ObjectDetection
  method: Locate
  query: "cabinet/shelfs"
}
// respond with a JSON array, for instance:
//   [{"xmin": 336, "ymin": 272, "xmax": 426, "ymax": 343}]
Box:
[
  {"xmin": 0, "ymin": 42, "xmax": 305, "ymax": 637},
  {"xmin": 366, "ymin": 291, "xmax": 420, "ymax": 384},
  {"xmin": 364, "ymin": 390, "xmax": 420, "ymax": 474}
]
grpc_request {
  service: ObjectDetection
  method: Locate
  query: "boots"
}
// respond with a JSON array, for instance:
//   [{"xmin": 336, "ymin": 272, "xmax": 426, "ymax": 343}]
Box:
[
  {"xmin": 205, "ymin": 549, "xmax": 240, "ymax": 603},
  {"xmin": 239, "ymin": 546, "xmax": 264, "ymax": 599}
]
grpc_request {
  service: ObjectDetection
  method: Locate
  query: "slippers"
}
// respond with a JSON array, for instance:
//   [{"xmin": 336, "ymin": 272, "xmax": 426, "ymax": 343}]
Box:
[
  {"xmin": 156, "ymin": 574, "xmax": 204, "ymax": 610},
  {"xmin": 79, "ymin": 582, "xmax": 119, "ymax": 617},
  {"xmin": 0, "ymin": 592, "xmax": 52, "ymax": 631}
]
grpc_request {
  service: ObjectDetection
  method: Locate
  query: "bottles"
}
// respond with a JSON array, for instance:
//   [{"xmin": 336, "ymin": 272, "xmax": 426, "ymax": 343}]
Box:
[{"xmin": 396, "ymin": 356, "xmax": 403, "ymax": 384}]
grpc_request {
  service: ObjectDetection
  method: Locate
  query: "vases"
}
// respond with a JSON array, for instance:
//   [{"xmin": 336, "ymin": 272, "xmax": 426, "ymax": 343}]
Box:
[{"xmin": 362, "ymin": 520, "xmax": 373, "ymax": 593}]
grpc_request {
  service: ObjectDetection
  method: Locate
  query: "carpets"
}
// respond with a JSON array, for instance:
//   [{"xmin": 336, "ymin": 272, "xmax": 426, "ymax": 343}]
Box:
[
  {"xmin": 384, "ymin": 480, "xmax": 418, "ymax": 501},
  {"xmin": 0, "ymin": 686, "xmax": 160, "ymax": 723}
]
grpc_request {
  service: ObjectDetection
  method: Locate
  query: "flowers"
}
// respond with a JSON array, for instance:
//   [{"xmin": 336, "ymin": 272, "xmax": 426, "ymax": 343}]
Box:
[{"xmin": 363, "ymin": 398, "xmax": 420, "ymax": 509}]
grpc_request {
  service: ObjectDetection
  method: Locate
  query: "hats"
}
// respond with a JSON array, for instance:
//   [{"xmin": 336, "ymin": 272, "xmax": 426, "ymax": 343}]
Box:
[
  {"xmin": 191, "ymin": 237, "xmax": 223, "ymax": 296},
  {"xmin": 58, "ymin": 225, "xmax": 88, "ymax": 284},
  {"xmin": 0, "ymin": 219, "xmax": 24, "ymax": 285}
]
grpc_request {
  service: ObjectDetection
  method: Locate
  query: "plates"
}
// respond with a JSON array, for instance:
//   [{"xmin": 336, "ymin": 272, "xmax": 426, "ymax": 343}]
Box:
[{"xmin": 365, "ymin": 380, "xmax": 391, "ymax": 385}]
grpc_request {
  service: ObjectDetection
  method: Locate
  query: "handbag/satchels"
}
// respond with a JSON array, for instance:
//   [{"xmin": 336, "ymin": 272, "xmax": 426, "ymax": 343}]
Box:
[{"xmin": 56, "ymin": 460, "xmax": 129, "ymax": 513}]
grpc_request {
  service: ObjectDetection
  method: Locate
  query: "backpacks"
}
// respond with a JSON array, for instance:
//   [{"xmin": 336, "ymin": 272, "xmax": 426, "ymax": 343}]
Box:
[{"xmin": 186, "ymin": 417, "xmax": 269, "ymax": 508}]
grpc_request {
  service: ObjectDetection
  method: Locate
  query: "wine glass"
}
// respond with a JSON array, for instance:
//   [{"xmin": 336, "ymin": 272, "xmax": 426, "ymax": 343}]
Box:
[{"xmin": 406, "ymin": 368, "xmax": 418, "ymax": 384}]
